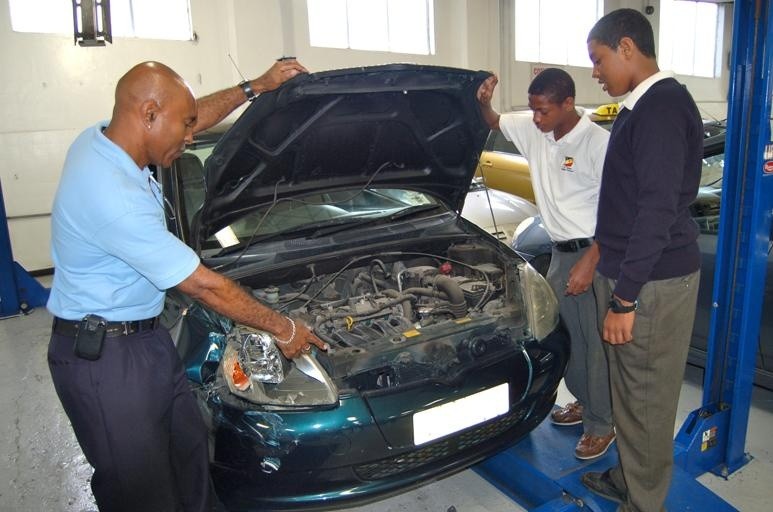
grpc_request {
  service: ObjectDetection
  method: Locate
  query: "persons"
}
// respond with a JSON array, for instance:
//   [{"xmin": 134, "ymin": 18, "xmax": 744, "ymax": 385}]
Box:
[
  {"xmin": 43, "ymin": 55, "xmax": 331, "ymax": 512},
  {"xmin": 475, "ymin": 65, "xmax": 618, "ymax": 465},
  {"xmin": 579, "ymin": 7, "xmax": 702, "ymax": 510}
]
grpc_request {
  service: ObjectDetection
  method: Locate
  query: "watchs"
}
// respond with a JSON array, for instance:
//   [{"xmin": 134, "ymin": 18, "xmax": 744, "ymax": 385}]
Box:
[{"xmin": 606, "ymin": 294, "xmax": 637, "ymax": 314}]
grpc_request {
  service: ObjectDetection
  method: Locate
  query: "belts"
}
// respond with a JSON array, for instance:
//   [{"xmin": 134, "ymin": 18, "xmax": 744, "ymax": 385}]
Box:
[
  {"xmin": 53, "ymin": 318, "xmax": 159, "ymax": 336},
  {"xmin": 553, "ymin": 236, "xmax": 596, "ymax": 252}
]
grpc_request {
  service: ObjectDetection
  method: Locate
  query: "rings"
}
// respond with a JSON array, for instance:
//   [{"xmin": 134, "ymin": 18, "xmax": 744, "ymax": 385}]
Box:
[{"xmin": 303, "ymin": 344, "xmax": 310, "ymax": 351}]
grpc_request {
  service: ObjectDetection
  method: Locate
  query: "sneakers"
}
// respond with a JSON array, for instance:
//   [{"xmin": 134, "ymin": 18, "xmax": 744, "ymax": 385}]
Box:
[{"xmin": 550, "ymin": 402, "xmax": 621, "ymax": 500}]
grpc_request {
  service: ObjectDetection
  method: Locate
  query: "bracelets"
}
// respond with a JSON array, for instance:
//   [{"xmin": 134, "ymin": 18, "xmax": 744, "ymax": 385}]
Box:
[
  {"xmin": 271, "ymin": 315, "xmax": 294, "ymax": 345},
  {"xmin": 236, "ymin": 78, "xmax": 258, "ymax": 106}
]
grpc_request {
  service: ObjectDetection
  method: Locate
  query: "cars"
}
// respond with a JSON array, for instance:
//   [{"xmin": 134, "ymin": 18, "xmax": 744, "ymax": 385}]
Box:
[
  {"xmin": 419, "ymin": 105, "xmax": 773, "ymax": 392},
  {"xmin": 137, "ymin": 62, "xmax": 573, "ymax": 510}
]
